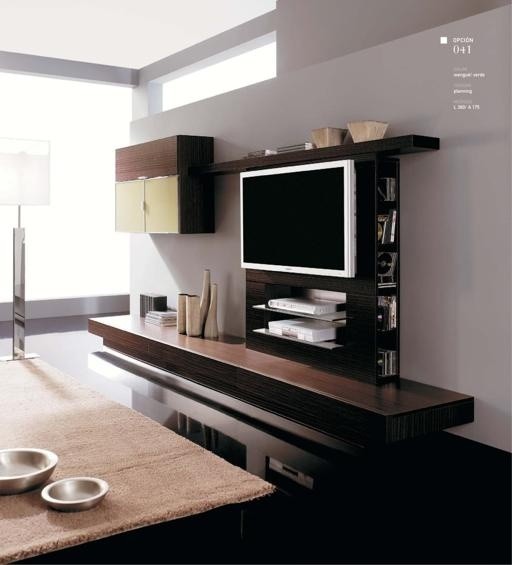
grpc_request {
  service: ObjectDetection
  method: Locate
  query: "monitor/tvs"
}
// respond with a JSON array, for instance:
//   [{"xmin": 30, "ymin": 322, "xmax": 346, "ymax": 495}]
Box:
[{"xmin": 240, "ymin": 159, "xmax": 357, "ymax": 278}]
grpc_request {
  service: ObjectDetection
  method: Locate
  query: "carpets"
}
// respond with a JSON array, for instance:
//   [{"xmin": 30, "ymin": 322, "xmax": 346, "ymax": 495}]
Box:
[{"xmin": 1, "ymin": 352, "xmax": 278, "ymax": 565}]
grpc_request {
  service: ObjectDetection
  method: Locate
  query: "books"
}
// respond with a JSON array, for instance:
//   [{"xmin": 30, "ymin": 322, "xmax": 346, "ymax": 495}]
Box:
[{"xmin": 138, "ymin": 292, "xmax": 177, "ymax": 327}]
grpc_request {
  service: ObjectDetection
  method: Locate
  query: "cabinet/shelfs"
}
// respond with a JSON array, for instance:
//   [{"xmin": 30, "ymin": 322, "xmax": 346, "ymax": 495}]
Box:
[
  {"xmin": 243, "ymin": 154, "xmax": 403, "ymax": 388},
  {"xmin": 113, "ymin": 134, "xmax": 215, "ymax": 236}
]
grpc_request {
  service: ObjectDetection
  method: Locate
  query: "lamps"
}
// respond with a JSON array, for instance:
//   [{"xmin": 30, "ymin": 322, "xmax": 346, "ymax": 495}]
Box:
[{"xmin": 0, "ymin": 137, "xmax": 55, "ymax": 363}]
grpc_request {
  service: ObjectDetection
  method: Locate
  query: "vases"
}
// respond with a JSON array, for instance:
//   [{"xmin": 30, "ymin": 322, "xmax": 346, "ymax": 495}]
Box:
[
  {"xmin": 200, "ymin": 269, "xmax": 210, "ymax": 325},
  {"xmin": 204, "ymin": 283, "xmax": 218, "ymax": 338},
  {"xmin": 185, "ymin": 294, "xmax": 202, "ymax": 336},
  {"xmin": 176, "ymin": 293, "xmax": 188, "ymax": 336}
]
